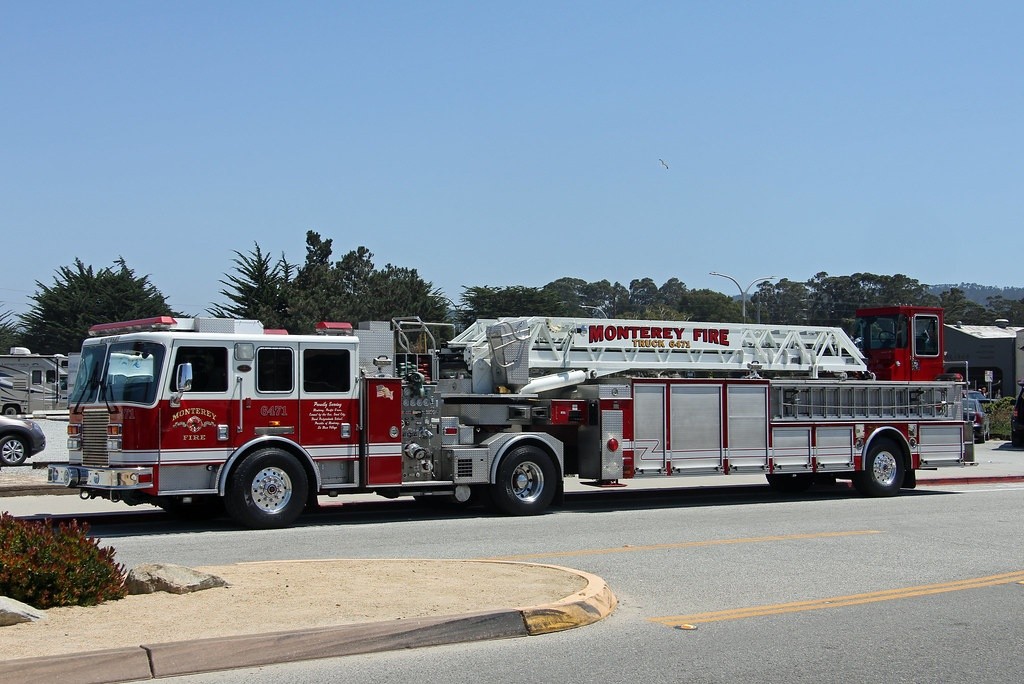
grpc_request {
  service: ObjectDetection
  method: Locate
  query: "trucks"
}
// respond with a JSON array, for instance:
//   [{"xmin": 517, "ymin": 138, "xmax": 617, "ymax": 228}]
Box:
[{"xmin": 0, "ymin": 346, "xmax": 192, "ymax": 417}]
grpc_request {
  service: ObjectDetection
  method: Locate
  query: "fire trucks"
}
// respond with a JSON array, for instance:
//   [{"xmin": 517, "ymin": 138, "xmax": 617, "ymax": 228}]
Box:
[{"xmin": 45, "ymin": 314, "xmax": 981, "ymax": 534}]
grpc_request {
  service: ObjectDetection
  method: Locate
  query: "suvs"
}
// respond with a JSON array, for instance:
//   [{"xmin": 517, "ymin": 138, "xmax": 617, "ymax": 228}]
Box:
[{"xmin": 1009, "ymin": 379, "xmax": 1024, "ymax": 449}]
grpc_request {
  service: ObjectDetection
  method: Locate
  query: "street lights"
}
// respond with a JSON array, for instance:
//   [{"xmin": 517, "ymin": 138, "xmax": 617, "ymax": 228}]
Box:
[{"xmin": 709, "ymin": 271, "xmax": 779, "ymax": 323}]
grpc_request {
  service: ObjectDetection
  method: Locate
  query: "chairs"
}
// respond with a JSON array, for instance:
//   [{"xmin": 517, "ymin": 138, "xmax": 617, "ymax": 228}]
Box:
[{"xmin": 121, "ymin": 378, "xmax": 148, "ymax": 401}]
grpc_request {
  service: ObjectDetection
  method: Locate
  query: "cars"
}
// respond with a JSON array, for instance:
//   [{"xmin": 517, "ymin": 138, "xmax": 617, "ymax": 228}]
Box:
[
  {"xmin": 0, "ymin": 415, "xmax": 47, "ymax": 467},
  {"xmin": 961, "ymin": 390, "xmax": 997, "ymax": 405},
  {"xmin": 962, "ymin": 398, "xmax": 992, "ymax": 444}
]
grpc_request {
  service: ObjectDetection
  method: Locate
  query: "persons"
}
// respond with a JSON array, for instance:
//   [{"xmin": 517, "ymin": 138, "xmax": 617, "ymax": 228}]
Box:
[{"xmin": 979, "ymin": 386, "xmax": 1003, "ymax": 400}]
